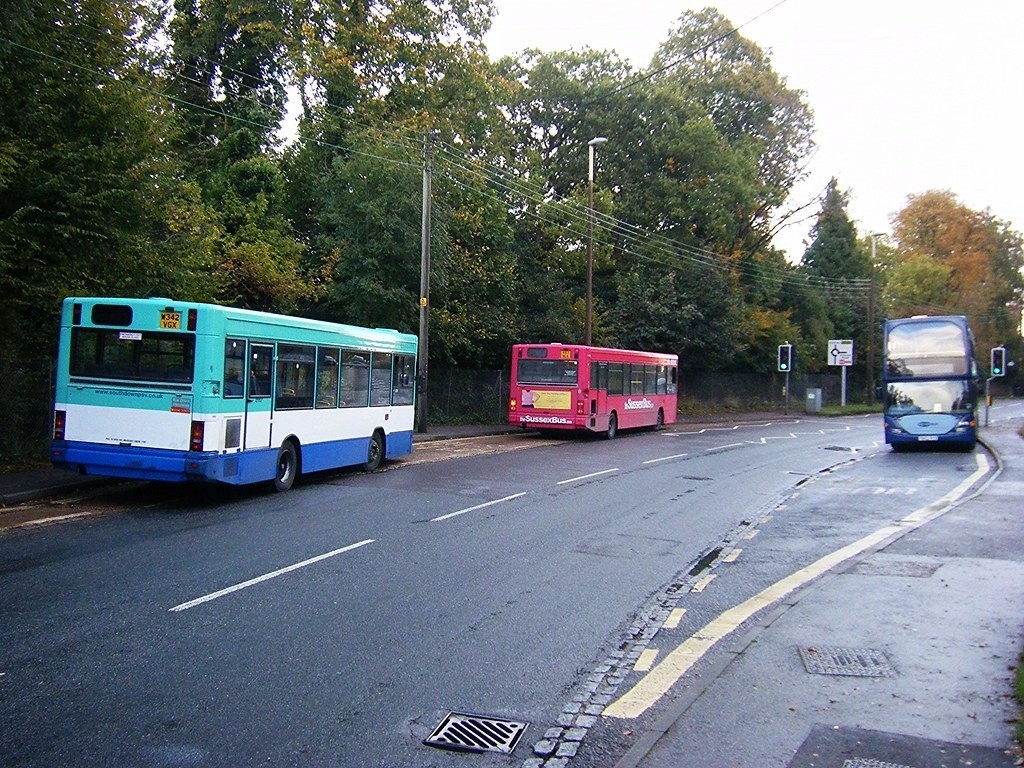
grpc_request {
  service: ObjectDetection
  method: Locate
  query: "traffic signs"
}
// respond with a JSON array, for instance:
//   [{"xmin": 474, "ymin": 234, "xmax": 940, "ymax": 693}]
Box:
[{"xmin": 826, "ymin": 340, "xmax": 853, "ymax": 367}]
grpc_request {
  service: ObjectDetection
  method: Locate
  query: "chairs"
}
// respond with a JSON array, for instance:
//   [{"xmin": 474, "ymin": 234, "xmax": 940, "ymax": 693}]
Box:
[{"xmin": 75, "ymin": 359, "xmax": 347, "ymax": 407}]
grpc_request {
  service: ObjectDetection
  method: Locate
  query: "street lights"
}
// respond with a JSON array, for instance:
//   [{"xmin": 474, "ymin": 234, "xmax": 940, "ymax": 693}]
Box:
[{"xmin": 584, "ymin": 137, "xmax": 609, "ymax": 346}]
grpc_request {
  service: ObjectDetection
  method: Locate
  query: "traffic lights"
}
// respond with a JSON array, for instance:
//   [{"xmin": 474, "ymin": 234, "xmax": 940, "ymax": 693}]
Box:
[
  {"xmin": 777, "ymin": 344, "xmax": 793, "ymax": 373},
  {"xmin": 990, "ymin": 348, "xmax": 1006, "ymax": 377}
]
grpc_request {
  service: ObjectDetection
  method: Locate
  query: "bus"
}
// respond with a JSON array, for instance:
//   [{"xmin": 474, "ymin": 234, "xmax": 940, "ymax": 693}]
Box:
[
  {"xmin": 509, "ymin": 344, "xmax": 680, "ymax": 439},
  {"xmin": 51, "ymin": 297, "xmax": 427, "ymax": 495},
  {"xmin": 882, "ymin": 314, "xmax": 980, "ymax": 453}
]
